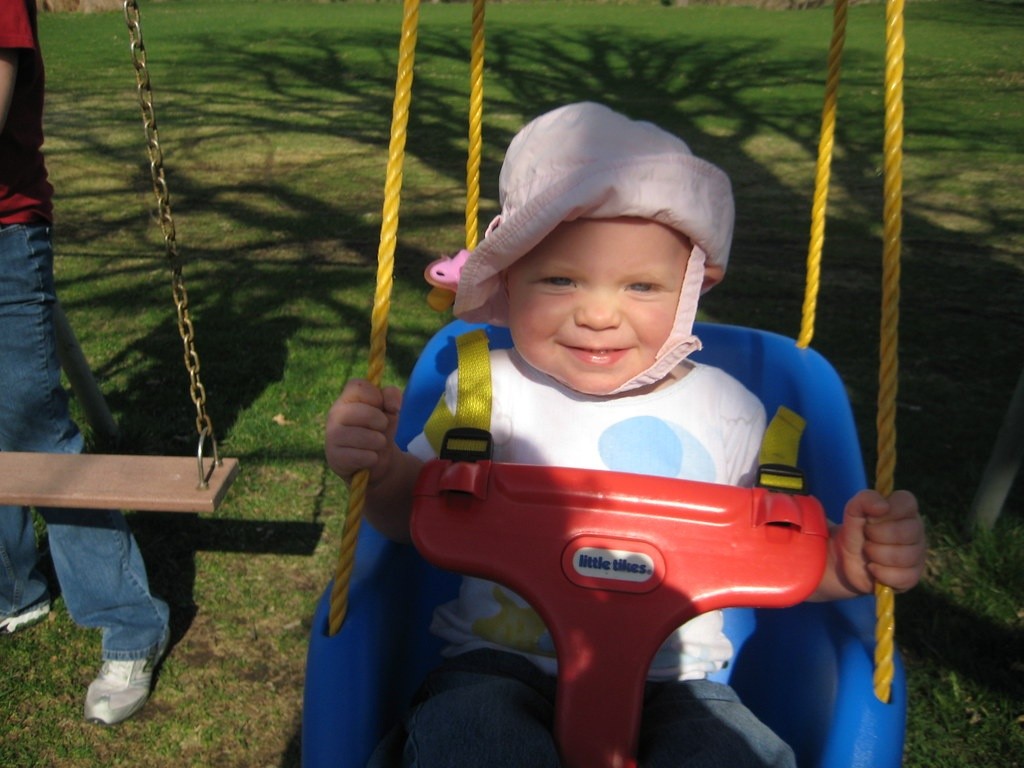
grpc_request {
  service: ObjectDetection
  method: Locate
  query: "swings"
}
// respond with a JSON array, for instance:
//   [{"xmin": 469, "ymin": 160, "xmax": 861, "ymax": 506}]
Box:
[
  {"xmin": 304, "ymin": 0, "xmax": 906, "ymax": 768},
  {"xmin": 0, "ymin": 0, "xmax": 238, "ymax": 511}
]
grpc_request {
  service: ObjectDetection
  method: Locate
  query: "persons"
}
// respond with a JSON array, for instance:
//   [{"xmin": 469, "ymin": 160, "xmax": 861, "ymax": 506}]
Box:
[
  {"xmin": 0, "ymin": 1, "xmax": 172, "ymax": 724},
  {"xmin": 323, "ymin": 103, "xmax": 926, "ymax": 768}
]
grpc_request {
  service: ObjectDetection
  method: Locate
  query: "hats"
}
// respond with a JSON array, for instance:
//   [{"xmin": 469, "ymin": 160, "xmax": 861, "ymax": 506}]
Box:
[{"xmin": 452, "ymin": 100, "xmax": 735, "ymax": 327}]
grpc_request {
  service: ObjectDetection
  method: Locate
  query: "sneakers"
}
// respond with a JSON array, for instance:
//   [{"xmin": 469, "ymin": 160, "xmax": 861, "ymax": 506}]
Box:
[
  {"xmin": 0, "ymin": 603, "xmax": 49, "ymax": 631},
  {"xmin": 84, "ymin": 625, "xmax": 170, "ymax": 725}
]
